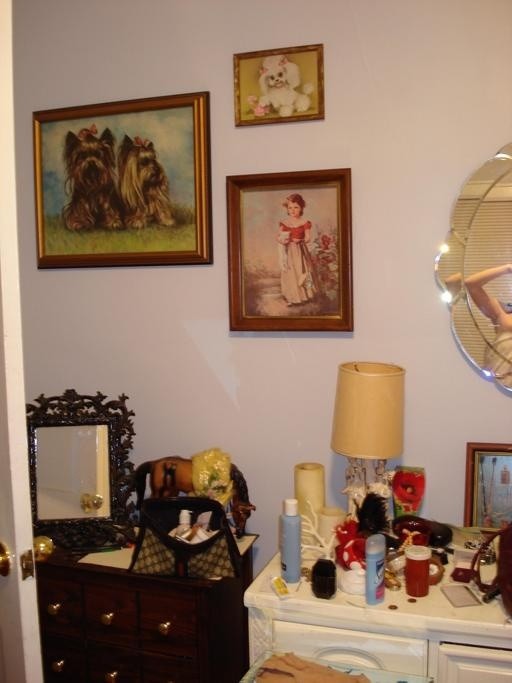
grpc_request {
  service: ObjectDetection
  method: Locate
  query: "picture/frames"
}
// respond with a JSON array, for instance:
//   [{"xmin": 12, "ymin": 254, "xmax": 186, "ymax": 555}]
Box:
[
  {"xmin": 233, "ymin": 43, "xmax": 325, "ymax": 127},
  {"xmin": 32, "ymin": 91, "xmax": 214, "ymax": 270},
  {"xmin": 225, "ymin": 168, "xmax": 352, "ymax": 333},
  {"xmin": 463, "ymin": 443, "xmax": 512, "ymax": 528}
]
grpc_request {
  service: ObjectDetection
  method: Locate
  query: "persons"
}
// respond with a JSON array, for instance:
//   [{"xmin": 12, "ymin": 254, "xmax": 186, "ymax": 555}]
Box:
[
  {"xmin": 445, "ymin": 272, "xmax": 463, "ymax": 297},
  {"xmin": 276, "ymin": 194, "xmax": 322, "ymax": 305},
  {"xmin": 463, "ymin": 262, "xmax": 512, "ymax": 389}
]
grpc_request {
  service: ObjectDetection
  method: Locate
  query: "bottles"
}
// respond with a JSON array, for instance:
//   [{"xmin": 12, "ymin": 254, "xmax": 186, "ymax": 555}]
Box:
[{"xmin": 404, "ymin": 544, "xmax": 432, "ymax": 597}]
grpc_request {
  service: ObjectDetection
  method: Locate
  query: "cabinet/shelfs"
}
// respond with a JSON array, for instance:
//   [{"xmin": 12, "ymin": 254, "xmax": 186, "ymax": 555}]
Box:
[
  {"xmin": 34, "ymin": 548, "xmax": 253, "ymax": 683},
  {"xmin": 436, "ymin": 642, "xmax": 512, "ymax": 683},
  {"xmin": 271, "ymin": 618, "xmax": 430, "ymax": 682}
]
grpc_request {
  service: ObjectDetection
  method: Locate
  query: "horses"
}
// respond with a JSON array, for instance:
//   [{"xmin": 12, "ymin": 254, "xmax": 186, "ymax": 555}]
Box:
[{"xmin": 135, "ymin": 455, "xmax": 257, "ymax": 539}]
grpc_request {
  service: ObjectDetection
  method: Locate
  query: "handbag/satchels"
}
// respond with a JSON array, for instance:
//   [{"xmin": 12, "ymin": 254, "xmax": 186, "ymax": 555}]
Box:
[{"xmin": 125, "ymin": 496, "xmax": 243, "ymax": 579}]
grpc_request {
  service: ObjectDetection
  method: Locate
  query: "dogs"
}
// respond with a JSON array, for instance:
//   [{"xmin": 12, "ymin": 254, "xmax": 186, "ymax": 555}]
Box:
[
  {"xmin": 258, "ymin": 54, "xmax": 316, "ymax": 118},
  {"xmin": 62, "ymin": 126, "xmax": 124, "ymax": 232},
  {"xmin": 116, "ymin": 133, "xmax": 176, "ymax": 230}
]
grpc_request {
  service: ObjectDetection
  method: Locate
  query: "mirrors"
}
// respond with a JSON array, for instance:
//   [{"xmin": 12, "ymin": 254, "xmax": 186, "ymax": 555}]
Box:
[
  {"xmin": 432, "ymin": 144, "xmax": 512, "ymax": 395},
  {"xmin": 27, "ymin": 389, "xmax": 134, "ymax": 550}
]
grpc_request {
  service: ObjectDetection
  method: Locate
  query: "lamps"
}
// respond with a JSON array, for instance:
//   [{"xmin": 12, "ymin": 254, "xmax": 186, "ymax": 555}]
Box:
[{"xmin": 331, "ymin": 361, "xmax": 406, "ymax": 519}]
